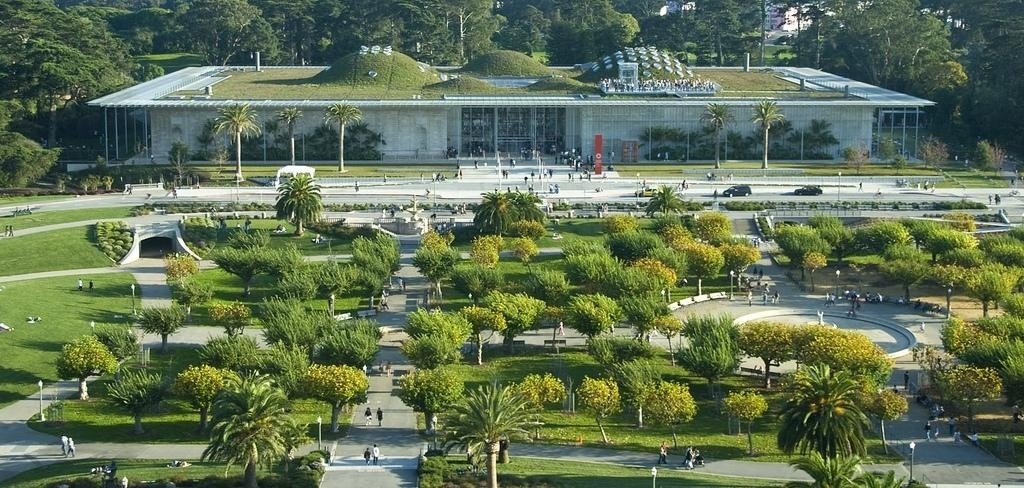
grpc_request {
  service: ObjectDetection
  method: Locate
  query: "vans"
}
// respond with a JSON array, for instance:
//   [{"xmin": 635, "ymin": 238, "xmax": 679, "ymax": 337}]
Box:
[{"xmin": 722, "ymin": 184, "xmax": 754, "ymax": 197}]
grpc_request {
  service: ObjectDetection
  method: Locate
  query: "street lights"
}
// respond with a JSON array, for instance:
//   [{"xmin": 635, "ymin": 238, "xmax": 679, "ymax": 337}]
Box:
[
  {"xmin": 330, "ymin": 294, "xmax": 334, "ymax": 317},
  {"xmin": 834, "ymin": 268, "xmax": 840, "ymax": 304},
  {"xmin": 649, "ymin": 466, "xmax": 658, "ymax": 487},
  {"xmin": 37, "ymin": 378, "xmax": 46, "ymax": 420},
  {"xmin": 635, "ymin": 169, "xmax": 641, "ymax": 205},
  {"xmin": 89, "ymin": 319, "xmax": 97, "ymax": 338},
  {"xmin": 909, "ymin": 441, "xmax": 916, "ymax": 482},
  {"xmin": 468, "ymin": 292, "xmax": 472, "ymax": 305},
  {"xmin": 837, "ymin": 170, "xmax": 842, "ymax": 200},
  {"xmin": 130, "ymin": 282, "xmax": 137, "ymax": 315},
  {"xmin": 660, "ymin": 288, "xmax": 666, "ymax": 303},
  {"xmin": 729, "ymin": 269, "xmax": 735, "ymax": 300},
  {"xmin": 432, "ymin": 415, "xmax": 439, "ymax": 449},
  {"xmin": 432, "ymin": 172, "xmax": 438, "ymax": 205},
  {"xmin": 376, "ymin": 223, "xmax": 382, "ymax": 236},
  {"xmin": 315, "ymin": 415, "xmax": 322, "ymax": 449}
]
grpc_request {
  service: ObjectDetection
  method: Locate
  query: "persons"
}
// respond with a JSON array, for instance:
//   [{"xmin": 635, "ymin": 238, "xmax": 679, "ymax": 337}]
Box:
[{"xmin": 4, "ymin": 78, "xmax": 1024, "ymax": 488}]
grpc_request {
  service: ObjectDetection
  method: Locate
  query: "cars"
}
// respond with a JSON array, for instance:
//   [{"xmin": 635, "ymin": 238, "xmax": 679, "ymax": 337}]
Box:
[
  {"xmin": 793, "ymin": 183, "xmax": 824, "ymax": 195},
  {"xmin": 634, "ymin": 184, "xmax": 665, "ymax": 197}
]
